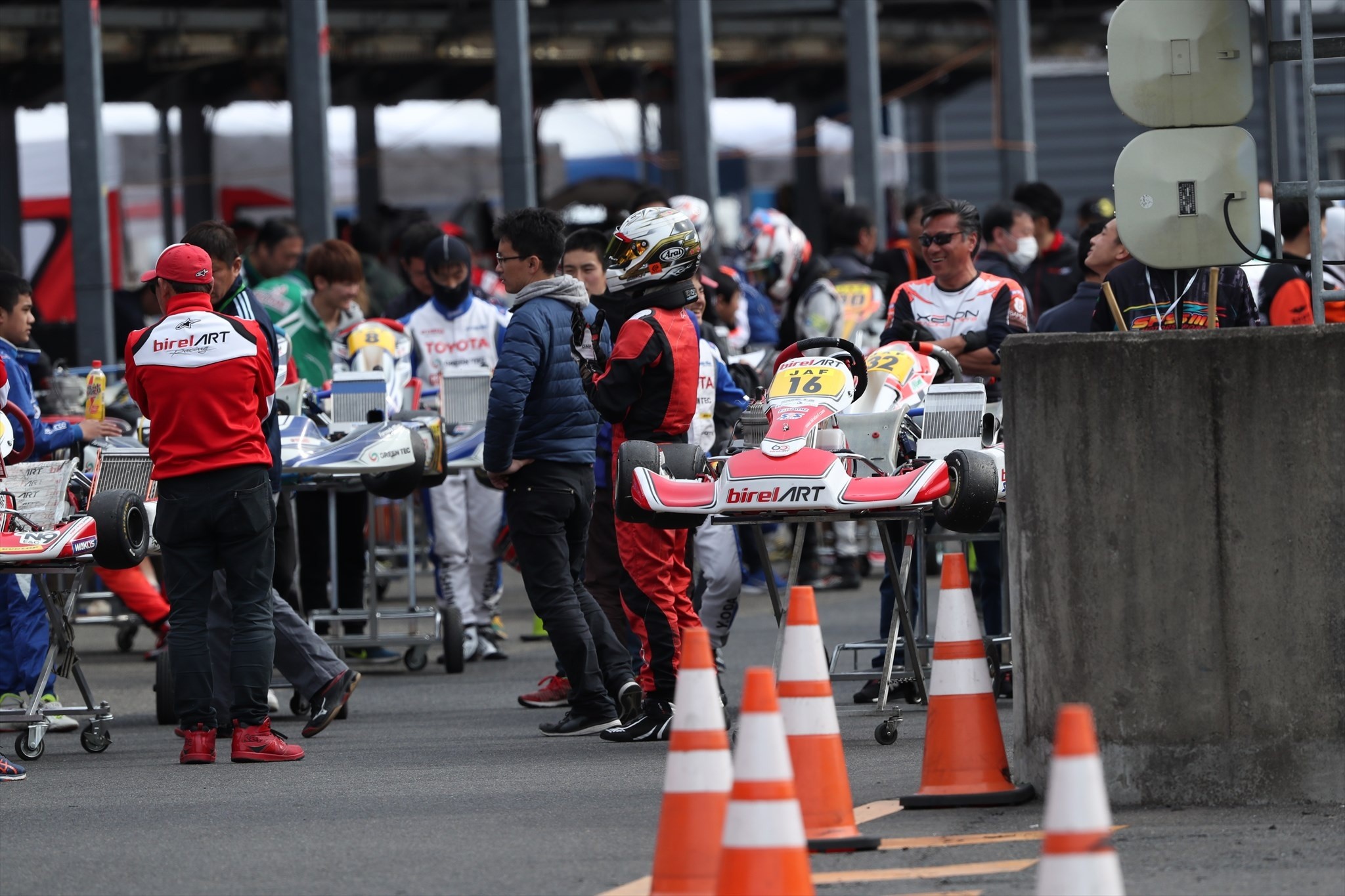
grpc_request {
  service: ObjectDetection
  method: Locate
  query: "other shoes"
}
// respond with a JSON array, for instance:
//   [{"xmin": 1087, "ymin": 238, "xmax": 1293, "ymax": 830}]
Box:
[
  {"xmin": 344, "ymin": 644, "xmax": 401, "ymax": 665},
  {"xmin": 301, "ymin": 669, "xmax": 361, "ymax": 738},
  {"xmin": 144, "ymin": 618, "xmax": 172, "ymax": 662},
  {"xmin": 267, "ymin": 689, "xmax": 279, "ymax": 714},
  {"xmin": 173, "ymin": 718, "xmax": 232, "ymax": 738},
  {"xmin": 739, "ymin": 564, "xmax": 788, "ymax": 595},
  {"xmin": 436, "ymin": 624, "xmax": 508, "ymax": 663},
  {"xmin": 854, "ymin": 680, "xmax": 911, "ymax": 704},
  {"xmin": 811, "ymin": 562, "xmax": 862, "ymax": 591},
  {"xmin": 998, "ymin": 672, "xmax": 1013, "ymax": 699},
  {"xmin": 0, "ymin": 694, "xmax": 29, "ymax": 731},
  {"xmin": 40, "ymin": 694, "xmax": 79, "ymax": 732}
]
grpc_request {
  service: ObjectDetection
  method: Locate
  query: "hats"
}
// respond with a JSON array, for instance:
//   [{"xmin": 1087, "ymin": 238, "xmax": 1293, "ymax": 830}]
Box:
[{"xmin": 141, "ymin": 242, "xmax": 214, "ymax": 284}]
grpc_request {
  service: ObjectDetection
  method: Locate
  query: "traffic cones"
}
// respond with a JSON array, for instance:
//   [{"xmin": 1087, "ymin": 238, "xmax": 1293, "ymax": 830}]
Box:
[
  {"xmin": 1035, "ymin": 703, "xmax": 1125, "ymax": 896},
  {"xmin": 713, "ymin": 667, "xmax": 814, "ymax": 896},
  {"xmin": 649, "ymin": 628, "xmax": 736, "ymax": 896},
  {"xmin": 898, "ymin": 552, "xmax": 1036, "ymax": 811},
  {"xmin": 778, "ymin": 584, "xmax": 881, "ymax": 854}
]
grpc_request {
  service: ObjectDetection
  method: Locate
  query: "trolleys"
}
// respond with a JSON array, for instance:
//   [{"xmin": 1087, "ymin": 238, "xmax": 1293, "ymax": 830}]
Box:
[
  {"xmin": 0, "ymin": 559, "xmax": 115, "ymax": 760},
  {"xmin": 822, "ymin": 508, "xmax": 1018, "ymax": 748}
]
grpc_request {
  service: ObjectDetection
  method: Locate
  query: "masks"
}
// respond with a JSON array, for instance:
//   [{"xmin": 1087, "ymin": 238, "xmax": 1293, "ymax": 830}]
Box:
[{"xmin": 999, "ymin": 229, "xmax": 1038, "ymax": 273}]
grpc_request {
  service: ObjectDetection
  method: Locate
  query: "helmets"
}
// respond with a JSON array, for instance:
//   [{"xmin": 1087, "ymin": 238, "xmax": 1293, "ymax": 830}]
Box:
[
  {"xmin": 668, "ymin": 195, "xmax": 715, "ymax": 255},
  {"xmin": 738, "ymin": 207, "xmax": 812, "ymax": 302},
  {"xmin": 603, "ymin": 207, "xmax": 701, "ymax": 293}
]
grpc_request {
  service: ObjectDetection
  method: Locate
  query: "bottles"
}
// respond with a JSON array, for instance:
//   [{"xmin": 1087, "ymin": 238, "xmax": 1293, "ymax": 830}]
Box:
[{"xmin": 85, "ymin": 360, "xmax": 105, "ymax": 423}]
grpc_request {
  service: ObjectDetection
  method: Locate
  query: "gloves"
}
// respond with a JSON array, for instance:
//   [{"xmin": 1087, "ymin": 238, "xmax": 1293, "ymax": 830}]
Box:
[
  {"xmin": 570, "ymin": 328, "xmax": 601, "ymax": 372},
  {"xmin": 570, "ymin": 302, "xmax": 607, "ymax": 366},
  {"xmin": 881, "ymin": 320, "xmax": 937, "ymax": 347}
]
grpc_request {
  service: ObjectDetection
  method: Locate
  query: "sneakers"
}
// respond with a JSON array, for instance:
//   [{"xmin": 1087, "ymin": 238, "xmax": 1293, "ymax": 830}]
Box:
[
  {"xmin": 517, "ymin": 676, "xmax": 570, "ymax": 707},
  {"xmin": 615, "ymin": 678, "xmax": 644, "ymax": 725},
  {"xmin": 599, "ymin": 699, "xmax": 676, "ymax": 742},
  {"xmin": 720, "ymin": 695, "xmax": 731, "ymax": 734},
  {"xmin": 538, "ymin": 707, "xmax": 621, "ymax": 737},
  {"xmin": 231, "ymin": 716, "xmax": 305, "ymax": 763},
  {"xmin": 0, "ymin": 745, "xmax": 27, "ymax": 781},
  {"xmin": 179, "ymin": 722, "xmax": 216, "ymax": 763}
]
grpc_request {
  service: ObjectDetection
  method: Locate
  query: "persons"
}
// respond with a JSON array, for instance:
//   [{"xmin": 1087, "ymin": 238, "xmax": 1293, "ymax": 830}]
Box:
[
  {"xmin": 482, "ymin": 209, "xmax": 642, "ymax": 736},
  {"xmin": 89, "ymin": 179, "xmax": 1345, "ymax": 706},
  {"xmin": 179, "ymin": 224, "xmax": 361, "ymax": 738},
  {"xmin": 0, "ymin": 271, "xmax": 122, "ymax": 731},
  {"xmin": 0, "ymin": 358, "xmax": 26, "ymax": 780},
  {"xmin": 570, "ymin": 207, "xmax": 732, "ymax": 743},
  {"xmin": 126, "ymin": 244, "xmax": 304, "ymax": 762}
]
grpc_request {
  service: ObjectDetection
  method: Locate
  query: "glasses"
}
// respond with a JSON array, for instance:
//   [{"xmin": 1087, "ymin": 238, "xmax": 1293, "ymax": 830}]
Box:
[
  {"xmin": 496, "ymin": 250, "xmax": 525, "ymax": 263},
  {"xmin": 918, "ymin": 229, "xmax": 966, "ymax": 248}
]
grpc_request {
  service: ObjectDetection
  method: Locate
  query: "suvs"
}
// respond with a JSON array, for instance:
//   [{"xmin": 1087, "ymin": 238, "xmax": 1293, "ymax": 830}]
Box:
[{"xmin": 20, "ymin": 185, "xmax": 296, "ymax": 328}]
